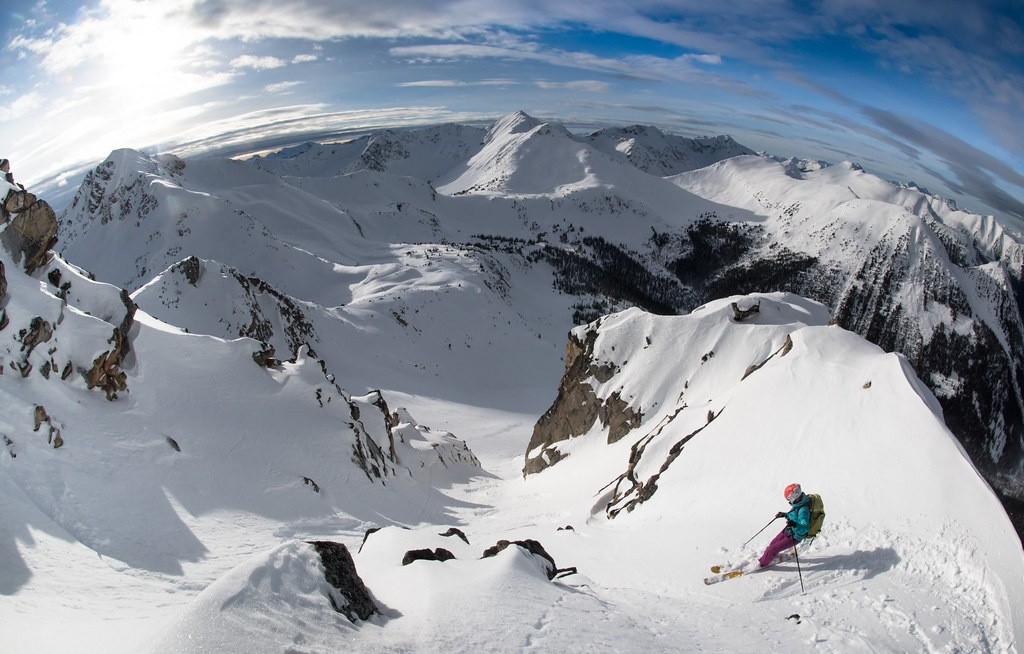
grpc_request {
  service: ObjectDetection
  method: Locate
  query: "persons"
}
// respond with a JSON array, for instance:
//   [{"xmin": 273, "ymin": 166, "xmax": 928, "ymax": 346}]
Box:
[{"xmin": 757, "ymin": 484, "xmax": 811, "ymax": 568}]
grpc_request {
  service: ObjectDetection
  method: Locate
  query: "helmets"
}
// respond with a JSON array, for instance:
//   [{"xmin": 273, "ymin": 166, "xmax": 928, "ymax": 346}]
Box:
[{"xmin": 784, "ymin": 484, "xmax": 802, "ymax": 503}]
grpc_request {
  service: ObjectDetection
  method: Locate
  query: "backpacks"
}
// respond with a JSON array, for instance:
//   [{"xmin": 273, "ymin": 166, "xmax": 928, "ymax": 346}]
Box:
[{"xmin": 796, "ymin": 494, "xmax": 825, "ymax": 545}]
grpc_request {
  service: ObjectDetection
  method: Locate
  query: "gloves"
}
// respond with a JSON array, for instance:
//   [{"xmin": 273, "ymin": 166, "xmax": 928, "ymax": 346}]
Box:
[
  {"xmin": 786, "ymin": 519, "xmax": 797, "ymax": 528},
  {"xmin": 775, "ymin": 512, "xmax": 786, "ymax": 518}
]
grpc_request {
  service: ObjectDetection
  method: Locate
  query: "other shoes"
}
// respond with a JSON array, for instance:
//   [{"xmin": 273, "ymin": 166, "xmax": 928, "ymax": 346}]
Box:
[{"xmin": 746, "ymin": 560, "xmax": 761, "ymax": 572}]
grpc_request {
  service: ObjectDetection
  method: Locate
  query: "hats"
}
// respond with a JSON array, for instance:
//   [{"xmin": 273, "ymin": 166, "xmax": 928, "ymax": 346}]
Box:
[{"xmin": 0, "ymin": 158, "xmax": 8, "ymax": 170}]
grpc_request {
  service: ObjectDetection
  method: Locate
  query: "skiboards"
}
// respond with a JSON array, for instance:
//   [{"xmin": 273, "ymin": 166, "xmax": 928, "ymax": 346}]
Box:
[{"xmin": 704, "ymin": 545, "xmax": 804, "ymax": 585}]
